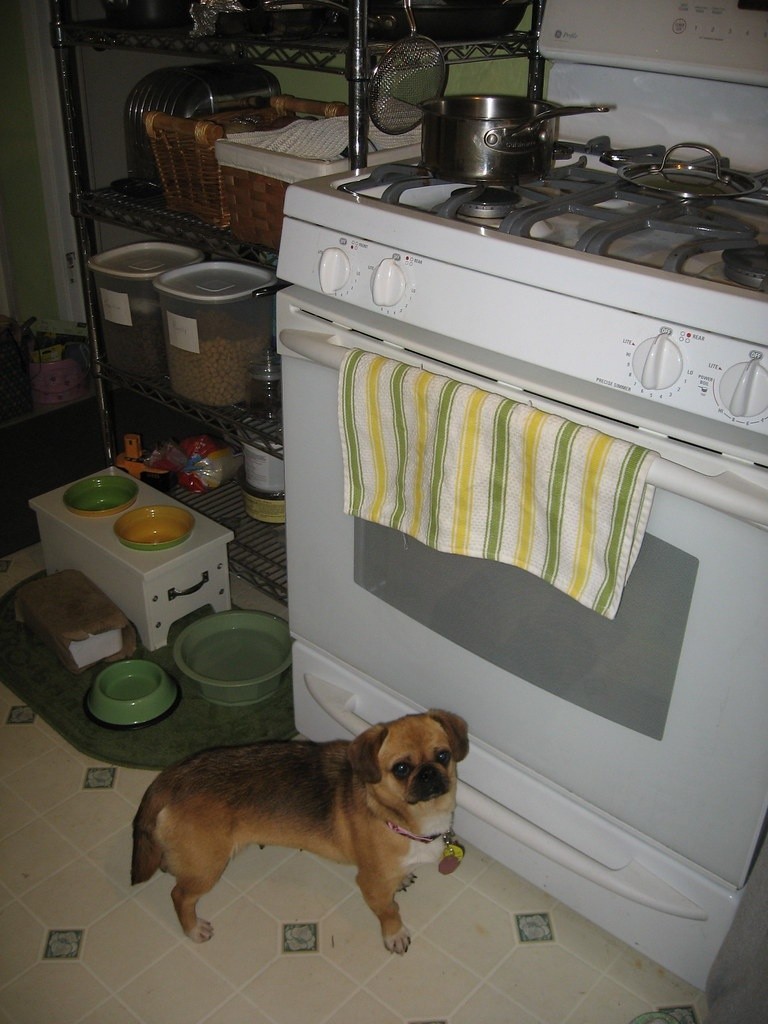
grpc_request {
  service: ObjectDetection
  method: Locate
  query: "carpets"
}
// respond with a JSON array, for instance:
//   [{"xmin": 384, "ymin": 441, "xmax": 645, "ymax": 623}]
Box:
[{"xmin": 0, "ymin": 568, "xmax": 300, "ymax": 772}]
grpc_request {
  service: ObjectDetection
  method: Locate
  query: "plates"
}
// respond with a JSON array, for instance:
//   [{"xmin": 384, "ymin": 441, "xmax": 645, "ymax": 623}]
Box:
[
  {"xmin": 63, "ymin": 477, "xmax": 140, "ymax": 515},
  {"xmin": 113, "ymin": 505, "xmax": 196, "ymax": 549}
]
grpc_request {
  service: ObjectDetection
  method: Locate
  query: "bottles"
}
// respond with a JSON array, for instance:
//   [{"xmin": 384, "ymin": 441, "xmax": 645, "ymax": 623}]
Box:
[{"xmin": 244, "ymin": 347, "xmax": 282, "ymax": 425}]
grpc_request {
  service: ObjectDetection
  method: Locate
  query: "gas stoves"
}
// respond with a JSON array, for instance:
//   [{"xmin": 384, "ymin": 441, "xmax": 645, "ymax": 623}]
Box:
[{"xmin": 275, "ymin": 0, "xmax": 768, "ymax": 354}]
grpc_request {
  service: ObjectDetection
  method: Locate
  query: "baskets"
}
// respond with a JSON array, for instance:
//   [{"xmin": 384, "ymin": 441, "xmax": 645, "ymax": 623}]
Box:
[
  {"xmin": 143, "ymin": 94, "xmax": 348, "ymax": 229},
  {"xmin": 214, "ymin": 139, "xmax": 421, "ymax": 250}
]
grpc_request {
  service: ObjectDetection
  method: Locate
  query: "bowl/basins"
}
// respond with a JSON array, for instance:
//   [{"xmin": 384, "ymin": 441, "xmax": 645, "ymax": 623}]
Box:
[
  {"xmin": 173, "ymin": 609, "xmax": 292, "ymax": 703},
  {"xmin": 83, "ymin": 660, "xmax": 176, "ymax": 725}
]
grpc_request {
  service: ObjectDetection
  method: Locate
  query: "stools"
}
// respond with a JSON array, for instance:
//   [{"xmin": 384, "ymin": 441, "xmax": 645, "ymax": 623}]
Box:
[{"xmin": 28, "ymin": 467, "xmax": 234, "ymax": 653}]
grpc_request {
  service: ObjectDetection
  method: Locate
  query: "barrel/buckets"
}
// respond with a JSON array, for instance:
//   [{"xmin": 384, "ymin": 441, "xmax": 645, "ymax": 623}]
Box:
[
  {"xmin": 236, "ymin": 416, "xmax": 285, "ymax": 523},
  {"xmin": 28, "ymin": 356, "xmax": 90, "ymax": 404}
]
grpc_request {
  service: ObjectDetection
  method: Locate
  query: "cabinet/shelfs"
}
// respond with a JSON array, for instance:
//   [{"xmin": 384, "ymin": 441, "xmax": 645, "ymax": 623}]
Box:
[{"xmin": 49, "ymin": 0, "xmax": 548, "ymax": 608}]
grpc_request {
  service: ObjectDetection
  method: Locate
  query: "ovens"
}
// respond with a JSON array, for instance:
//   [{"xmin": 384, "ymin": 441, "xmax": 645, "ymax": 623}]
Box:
[{"xmin": 277, "ymin": 287, "xmax": 768, "ymax": 891}]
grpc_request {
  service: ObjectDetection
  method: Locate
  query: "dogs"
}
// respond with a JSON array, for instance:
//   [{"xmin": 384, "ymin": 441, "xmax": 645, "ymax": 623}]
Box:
[{"xmin": 130, "ymin": 707, "xmax": 470, "ymax": 956}]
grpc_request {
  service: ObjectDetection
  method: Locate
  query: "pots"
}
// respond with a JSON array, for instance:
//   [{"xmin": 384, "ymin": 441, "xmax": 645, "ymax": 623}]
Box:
[
  {"xmin": 418, "ymin": 92, "xmax": 612, "ymax": 180},
  {"xmin": 93, "ymin": 0, "xmax": 535, "ymax": 40}
]
